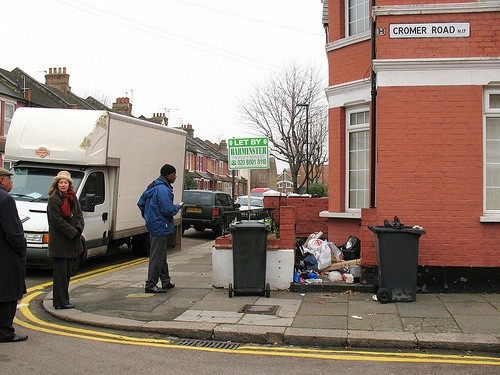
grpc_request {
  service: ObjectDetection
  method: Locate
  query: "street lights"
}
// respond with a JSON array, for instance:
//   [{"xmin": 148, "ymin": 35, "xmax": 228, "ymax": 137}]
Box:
[
  {"xmin": 296, "ymin": 103, "xmax": 309, "ymax": 194},
  {"xmin": 282, "ymin": 168, "xmax": 287, "ymax": 193}
]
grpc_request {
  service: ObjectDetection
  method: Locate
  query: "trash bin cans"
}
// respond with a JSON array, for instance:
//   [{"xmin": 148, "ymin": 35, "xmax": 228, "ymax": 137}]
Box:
[
  {"xmin": 229, "ymin": 221, "xmax": 271, "ymax": 298},
  {"xmin": 368, "ymin": 226, "xmax": 426, "ymax": 304}
]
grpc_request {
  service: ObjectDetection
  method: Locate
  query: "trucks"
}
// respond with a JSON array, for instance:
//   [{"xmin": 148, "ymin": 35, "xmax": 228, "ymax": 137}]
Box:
[{"xmin": 3, "ymin": 106, "xmax": 189, "ymax": 277}]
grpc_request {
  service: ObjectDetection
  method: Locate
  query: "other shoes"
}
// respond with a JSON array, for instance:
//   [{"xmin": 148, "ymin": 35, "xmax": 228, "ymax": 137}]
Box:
[
  {"xmin": 8, "ymin": 334, "xmax": 28, "ymax": 342},
  {"xmin": 145, "ymin": 285, "xmax": 167, "ymax": 293},
  {"xmin": 55, "ymin": 304, "xmax": 75, "ymax": 309},
  {"xmin": 162, "ymin": 283, "xmax": 175, "ymax": 290}
]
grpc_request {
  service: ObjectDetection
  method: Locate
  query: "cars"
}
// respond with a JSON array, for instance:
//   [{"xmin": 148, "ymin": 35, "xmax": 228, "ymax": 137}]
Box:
[{"xmin": 233, "ymin": 187, "xmax": 287, "ymax": 220}]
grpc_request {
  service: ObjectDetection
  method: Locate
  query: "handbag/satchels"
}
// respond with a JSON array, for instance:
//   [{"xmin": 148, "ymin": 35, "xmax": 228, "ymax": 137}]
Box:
[{"xmin": 79, "ymin": 235, "xmax": 88, "ymax": 262}]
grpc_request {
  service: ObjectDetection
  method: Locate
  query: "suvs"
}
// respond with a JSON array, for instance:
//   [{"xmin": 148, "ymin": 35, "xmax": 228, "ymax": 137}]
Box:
[{"xmin": 181, "ymin": 189, "xmax": 241, "ymax": 237}]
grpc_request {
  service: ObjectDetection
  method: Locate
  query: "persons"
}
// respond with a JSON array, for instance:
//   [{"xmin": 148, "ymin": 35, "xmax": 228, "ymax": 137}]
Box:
[
  {"xmin": 0, "ymin": 168, "xmax": 28, "ymax": 342},
  {"xmin": 47, "ymin": 171, "xmax": 85, "ymax": 309},
  {"xmin": 137, "ymin": 164, "xmax": 183, "ymax": 294}
]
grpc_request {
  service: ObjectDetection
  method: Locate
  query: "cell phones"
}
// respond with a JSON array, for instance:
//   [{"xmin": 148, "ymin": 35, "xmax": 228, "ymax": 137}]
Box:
[{"xmin": 179, "ymin": 202, "xmax": 184, "ymax": 206}]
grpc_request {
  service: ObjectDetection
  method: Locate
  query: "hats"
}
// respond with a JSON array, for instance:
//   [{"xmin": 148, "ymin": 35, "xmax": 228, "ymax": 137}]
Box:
[
  {"xmin": 160, "ymin": 164, "xmax": 176, "ymax": 176},
  {"xmin": 55, "ymin": 171, "xmax": 73, "ymax": 183},
  {"xmin": 0, "ymin": 167, "xmax": 14, "ymax": 175}
]
